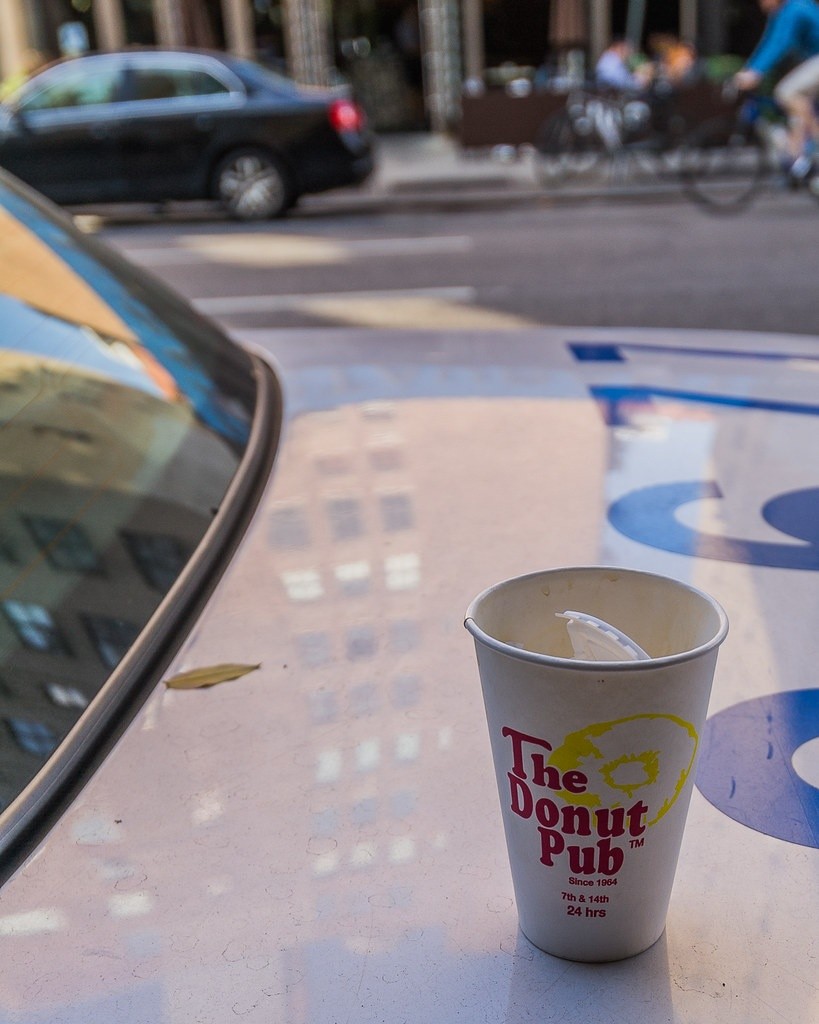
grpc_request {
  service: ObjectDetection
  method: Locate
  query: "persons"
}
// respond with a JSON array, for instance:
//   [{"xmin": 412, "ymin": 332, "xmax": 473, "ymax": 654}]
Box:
[
  {"xmin": 731, "ymin": 0, "xmax": 819, "ymax": 192},
  {"xmin": 594, "ymin": 38, "xmax": 653, "ymax": 92},
  {"xmin": 649, "ymin": 35, "xmax": 697, "ymax": 91}
]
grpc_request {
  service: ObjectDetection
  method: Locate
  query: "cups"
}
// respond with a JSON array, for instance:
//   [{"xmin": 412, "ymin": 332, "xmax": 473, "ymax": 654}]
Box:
[{"xmin": 463, "ymin": 565, "xmax": 729, "ymax": 965}]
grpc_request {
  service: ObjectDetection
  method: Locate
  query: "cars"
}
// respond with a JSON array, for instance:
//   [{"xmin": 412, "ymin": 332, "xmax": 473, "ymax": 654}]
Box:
[
  {"xmin": 0, "ymin": 46, "xmax": 373, "ymax": 222},
  {"xmin": 3, "ymin": 163, "xmax": 819, "ymax": 1021}
]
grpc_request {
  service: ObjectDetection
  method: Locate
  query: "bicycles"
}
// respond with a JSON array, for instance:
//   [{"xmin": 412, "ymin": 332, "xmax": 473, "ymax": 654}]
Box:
[{"xmin": 680, "ymin": 75, "xmax": 819, "ymax": 215}]
